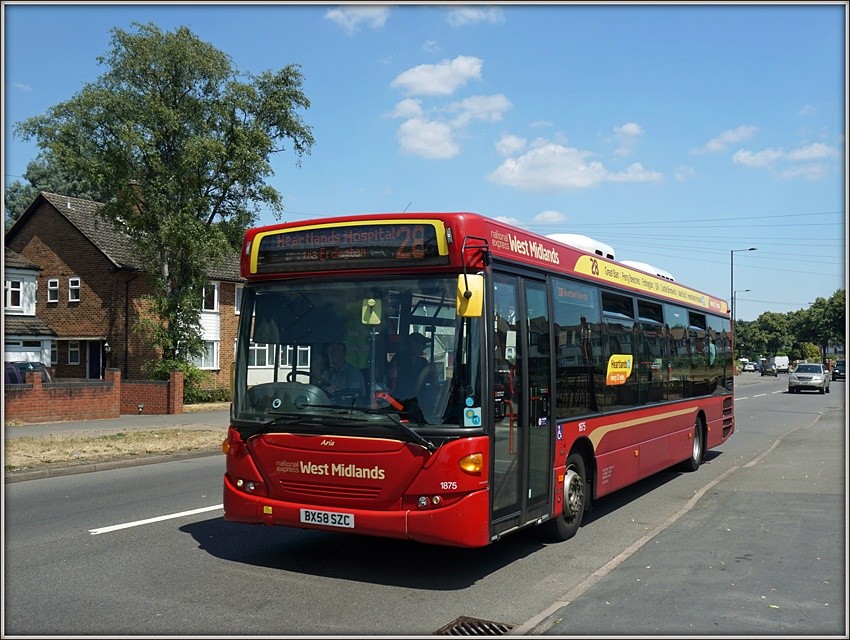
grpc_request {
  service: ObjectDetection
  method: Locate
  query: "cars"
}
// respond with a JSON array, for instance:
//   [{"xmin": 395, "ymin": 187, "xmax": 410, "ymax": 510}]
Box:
[
  {"xmin": 736, "ymin": 355, "xmax": 812, "ymax": 377},
  {"xmin": 788, "ymin": 363, "xmax": 830, "ymax": 394},
  {"xmin": 830, "ymin": 359, "xmax": 846, "ymax": 381}
]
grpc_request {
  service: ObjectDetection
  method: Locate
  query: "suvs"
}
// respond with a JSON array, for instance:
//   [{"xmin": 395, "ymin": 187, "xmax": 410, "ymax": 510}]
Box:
[{"xmin": 4, "ymin": 360, "xmax": 56, "ymax": 385}]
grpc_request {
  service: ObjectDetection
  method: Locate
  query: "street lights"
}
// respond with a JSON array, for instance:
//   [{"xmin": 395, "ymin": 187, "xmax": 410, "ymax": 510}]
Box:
[
  {"xmin": 734, "ymin": 289, "xmax": 752, "ymax": 363},
  {"xmin": 730, "ymin": 247, "xmax": 758, "ymax": 321}
]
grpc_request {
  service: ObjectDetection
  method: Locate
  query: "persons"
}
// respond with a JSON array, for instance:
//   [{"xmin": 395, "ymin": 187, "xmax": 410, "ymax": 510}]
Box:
[
  {"xmin": 530, "ymin": 334, "xmax": 563, "ymax": 387},
  {"xmin": 703, "ymin": 336, "xmax": 716, "ymax": 365},
  {"xmin": 319, "ymin": 342, "xmax": 366, "ymax": 406},
  {"xmin": 387, "ymin": 332, "xmax": 431, "ymax": 403}
]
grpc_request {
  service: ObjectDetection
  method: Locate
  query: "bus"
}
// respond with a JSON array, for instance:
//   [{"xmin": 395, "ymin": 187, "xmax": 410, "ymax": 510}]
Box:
[{"xmin": 221, "ymin": 211, "xmax": 735, "ymax": 551}]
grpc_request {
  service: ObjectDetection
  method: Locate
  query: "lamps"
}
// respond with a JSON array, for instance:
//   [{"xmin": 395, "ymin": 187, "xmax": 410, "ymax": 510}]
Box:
[{"xmin": 104, "ymin": 342, "xmax": 111, "ymax": 354}]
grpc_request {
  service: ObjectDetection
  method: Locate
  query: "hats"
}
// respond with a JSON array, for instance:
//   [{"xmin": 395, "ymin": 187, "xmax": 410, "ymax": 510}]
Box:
[{"xmin": 408, "ymin": 332, "xmax": 431, "ymax": 343}]
